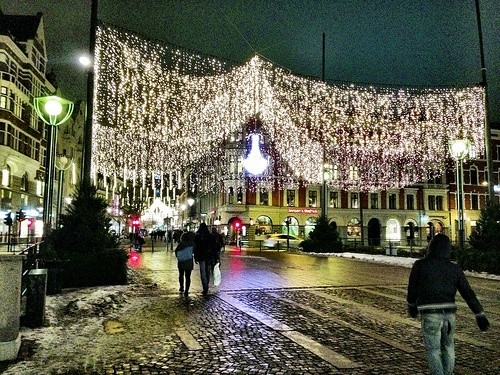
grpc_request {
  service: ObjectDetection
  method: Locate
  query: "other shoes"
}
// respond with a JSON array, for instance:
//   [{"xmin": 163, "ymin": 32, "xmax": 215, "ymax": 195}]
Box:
[
  {"xmin": 184, "ymin": 292, "xmax": 188, "ymax": 297},
  {"xmin": 202, "ymin": 290, "xmax": 209, "ymax": 298},
  {"xmin": 179, "ymin": 287, "xmax": 184, "ymax": 292}
]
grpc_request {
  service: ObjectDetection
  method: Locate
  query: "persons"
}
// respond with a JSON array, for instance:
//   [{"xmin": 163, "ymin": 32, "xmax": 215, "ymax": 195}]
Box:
[
  {"xmin": 210, "ymin": 227, "xmax": 223, "ymax": 274},
  {"xmin": 194, "ymin": 223, "xmax": 216, "ymax": 295},
  {"xmin": 407, "ymin": 233, "xmax": 490, "ymax": 375},
  {"xmin": 175, "ymin": 233, "xmax": 194, "ymax": 294}
]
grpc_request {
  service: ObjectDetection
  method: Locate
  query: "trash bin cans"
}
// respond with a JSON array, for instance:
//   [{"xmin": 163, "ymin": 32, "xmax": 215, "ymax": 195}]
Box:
[{"xmin": 25, "ymin": 269, "xmax": 48, "ymax": 328}]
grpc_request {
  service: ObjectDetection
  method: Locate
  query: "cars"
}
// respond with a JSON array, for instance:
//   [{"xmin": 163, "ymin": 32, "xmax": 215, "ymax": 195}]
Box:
[
  {"xmin": 109, "ymin": 230, "xmax": 146, "ymax": 253},
  {"xmin": 264, "ymin": 233, "xmax": 303, "ymax": 249}
]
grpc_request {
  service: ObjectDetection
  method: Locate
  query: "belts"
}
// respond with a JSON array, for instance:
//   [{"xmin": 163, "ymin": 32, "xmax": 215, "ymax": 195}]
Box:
[{"xmin": 421, "ymin": 308, "xmax": 456, "ymax": 315}]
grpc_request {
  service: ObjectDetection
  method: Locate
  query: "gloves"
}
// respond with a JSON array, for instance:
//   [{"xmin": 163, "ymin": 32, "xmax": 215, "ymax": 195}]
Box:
[
  {"xmin": 476, "ymin": 315, "xmax": 490, "ymax": 332},
  {"xmin": 408, "ymin": 304, "xmax": 419, "ymax": 318}
]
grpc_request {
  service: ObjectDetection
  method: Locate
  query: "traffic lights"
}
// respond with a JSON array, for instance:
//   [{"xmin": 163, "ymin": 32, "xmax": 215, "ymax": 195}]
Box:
[
  {"xmin": 234, "ymin": 221, "xmax": 240, "ymax": 228},
  {"xmin": 4, "ymin": 213, "xmax": 13, "ymax": 226},
  {"xmin": 132, "ymin": 219, "xmax": 139, "ymax": 225},
  {"xmin": 16, "ymin": 210, "xmax": 27, "ymax": 221}
]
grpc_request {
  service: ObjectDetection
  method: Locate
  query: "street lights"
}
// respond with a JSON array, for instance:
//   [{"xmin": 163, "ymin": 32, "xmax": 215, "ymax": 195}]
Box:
[
  {"xmin": 55, "ymin": 155, "xmax": 74, "ymax": 229},
  {"xmin": 180, "ymin": 202, "xmax": 188, "ymax": 240},
  {"xmin": 187, "ymin": 197, "xmax": 195, "ymax": 237},
  {"xmin": 34, "ymin": 95, "xmax": 74, "ymax": 233},
  {"xmin": 448, "ymin": 139, "xmax": 470, "ymax": 247}
]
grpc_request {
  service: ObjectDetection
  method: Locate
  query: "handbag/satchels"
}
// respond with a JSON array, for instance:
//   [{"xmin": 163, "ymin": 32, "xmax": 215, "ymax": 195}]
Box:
[{"xmin": 212, "ymin": 263, "xmax": 221, "ymax": 286}]
